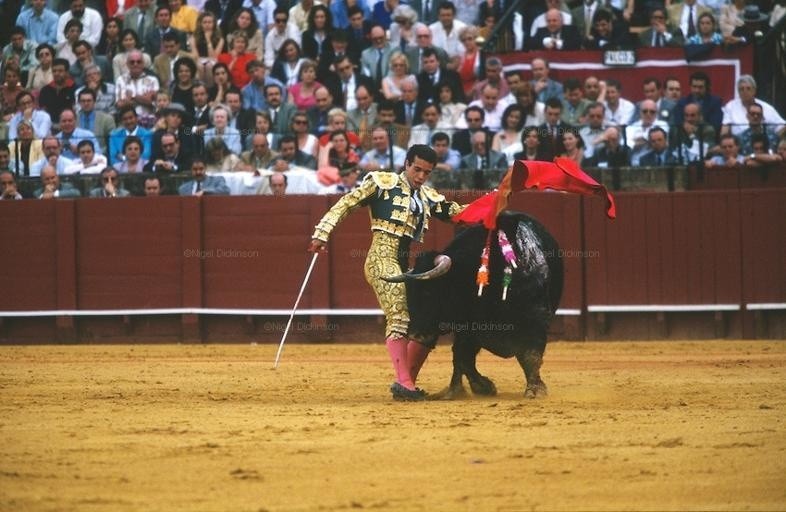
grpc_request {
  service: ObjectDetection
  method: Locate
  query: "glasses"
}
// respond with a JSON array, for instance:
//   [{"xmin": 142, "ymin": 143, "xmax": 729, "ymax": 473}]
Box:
[{"xmin": 1, "ymin": 16, "xmax": 765, "ymax": 184}]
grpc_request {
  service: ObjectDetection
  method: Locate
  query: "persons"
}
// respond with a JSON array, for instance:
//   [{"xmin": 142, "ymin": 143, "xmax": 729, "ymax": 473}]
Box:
[
  {"xmin": 2, "ymin": 0, "xmax": 786, "ymax": 202},
  {"xmin": 306, "ymin": 144, "xmax": 471, "ymax": 401}
]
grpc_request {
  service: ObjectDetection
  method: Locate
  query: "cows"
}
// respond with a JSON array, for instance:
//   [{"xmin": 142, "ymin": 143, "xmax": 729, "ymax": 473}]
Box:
[{"xmin": 379, "ymin": 210, "xmax": 564, "ymax": 398}]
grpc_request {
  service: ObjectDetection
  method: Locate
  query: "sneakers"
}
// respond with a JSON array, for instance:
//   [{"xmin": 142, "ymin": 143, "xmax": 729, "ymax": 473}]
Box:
[{"xmin": 389, "ymin": 380, "xmax": 430, "ymax": 402}]
